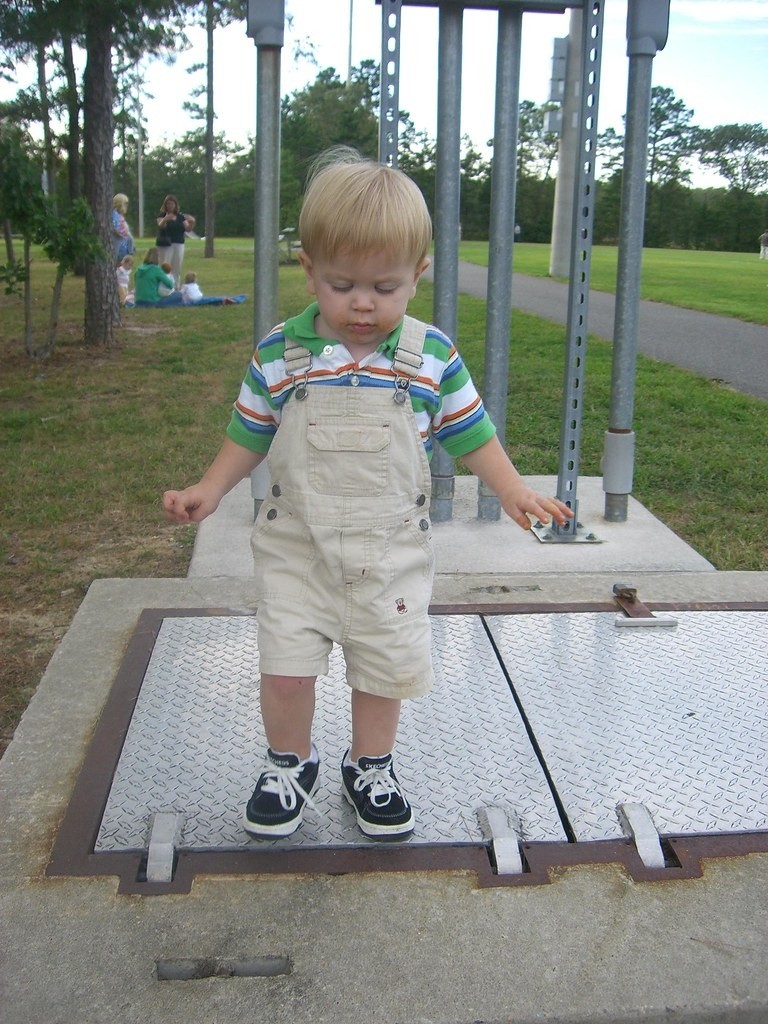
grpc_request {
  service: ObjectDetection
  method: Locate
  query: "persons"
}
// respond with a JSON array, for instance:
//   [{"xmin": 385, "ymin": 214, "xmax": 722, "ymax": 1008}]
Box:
[
  {"xmin": 133, "ymin": 247, "xmax": 182, "ymax": 307},
  {"xmin": 116, "ymin": 255, "xmax": 134, "ymax": 305},
  {"xmin": 758, "ymin": 230, "xmax": 768, "ymax": 259},
  {"xmin": 179, "ymin": 273, "xmax": 203, "ymax": 302},
  {"xmin": 162, "ymin": 161, "xmax": 574, "ymax": 835},
  {"xmin": 112, "ymin": 192, "xmax": 136, "ymax": 260},
  {"xmin": 514, "ymin": 223, "xmax": 521, "ymax": 242},
  {"xmin": 156, "ymin": 194, "xmax": 195, "ymax": 291}
]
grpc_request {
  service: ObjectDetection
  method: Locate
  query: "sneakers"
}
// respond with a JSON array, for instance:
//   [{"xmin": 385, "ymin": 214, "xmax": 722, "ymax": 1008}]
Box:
[
  {"xmin": 241, "ymin": 743, "xmax": 321, "ymax": 836},
  {"xmin": 340, "ymin": 747, "xmax": 416, "ymax": 838}
]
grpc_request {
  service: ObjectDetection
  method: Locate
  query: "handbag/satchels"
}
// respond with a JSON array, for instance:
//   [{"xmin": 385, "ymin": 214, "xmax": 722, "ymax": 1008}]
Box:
[{"xmin": 156, "ymin": 228, "xmax": 171, "ymax": 246}]
